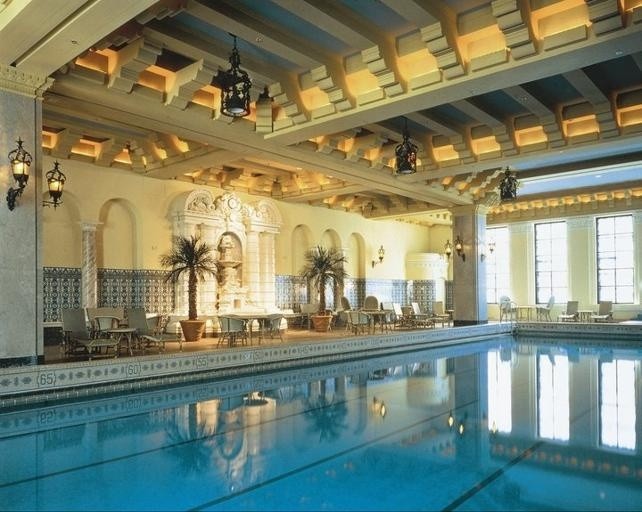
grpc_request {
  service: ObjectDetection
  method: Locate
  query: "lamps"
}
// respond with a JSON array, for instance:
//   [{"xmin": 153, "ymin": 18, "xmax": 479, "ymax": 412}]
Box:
[
  {"xmin": 443, "ymin": 238, "xmax": 453, "ymax": 260},
  {"xmin": 395, "ymin": 119, "xmax": 417, "ymax": 174},
  {"xmin": 502, "ymin": 165, "xmax": 518, "ymax": 203},
  {"xmin": 454, "ymin": 233, "xmax": 467, "ymax": 264},
  {"xmin": 448, "ymin": 407, "xmax": 455, "ymax": 433},
  {"xmin": 458, "ymin": 414, "xmax": 468, "ymax": 440},
  {"xmin": 371, "ymin": 396, "xmax": 386, "ymax": 420},
  {"xmin": 7, "ymin": 136, "xmax": 33, "ymax": 210},
  {"xmin": 221, "ymin": 30, "xmax": 250, "ymax": 119},
  {"xmin": 372, "ymin": 244, "xmax": 385, "ymax": 267},
  {"xmin": 481, "ymin": 239, "xmax": 497, "ymax": 262},
  {"xmin": 491, "ymin": 422, "xmax": 498, "ymax": 440},
  {"xmin": 42, "ymin": 157, "xmax": 68, "ymax": 210}
]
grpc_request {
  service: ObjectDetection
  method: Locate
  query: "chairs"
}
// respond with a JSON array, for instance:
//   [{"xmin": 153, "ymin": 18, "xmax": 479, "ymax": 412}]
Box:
[
  {"xmin": 210, "ymin": 305, "xmax": 297, "ymax": 349},
  {"xmin": 500, "ymin": 343, "xmax": 614, "ymax": 363},
  {"xmin": 339, "ymin": 295, "xmax": 453, "ymax": 334},
  {"xmin": 58, "ymin": 300, "xmax": 187, "ymax": 360},
  {"xmin": 500, "ymin": 292, "xmax": 615, "ymax": 322}
]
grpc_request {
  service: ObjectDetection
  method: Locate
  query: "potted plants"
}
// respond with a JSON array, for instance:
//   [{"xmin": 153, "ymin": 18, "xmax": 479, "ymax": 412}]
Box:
[
  {"xmin": 302, "ymin": 243, "xmax": 347, "ymax": 334},
  {"xmin": 158, "ymin": 233, "xmax": 218, "ymax": 341}
]
